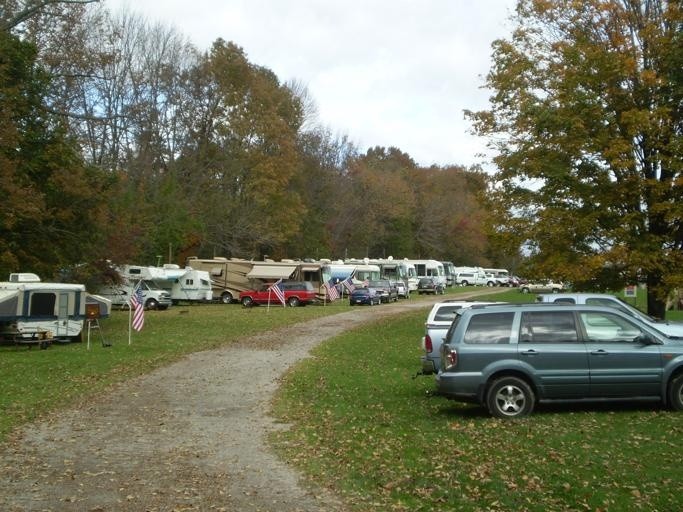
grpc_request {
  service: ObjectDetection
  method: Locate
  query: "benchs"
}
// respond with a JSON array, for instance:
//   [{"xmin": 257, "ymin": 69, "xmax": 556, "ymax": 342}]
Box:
[{"xmin": 1, "ymin": 339, "xmax": 48, "ymax": 344}]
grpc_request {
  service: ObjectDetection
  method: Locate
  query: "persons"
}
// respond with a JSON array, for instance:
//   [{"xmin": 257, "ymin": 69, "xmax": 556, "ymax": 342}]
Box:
[{"xmin": 361, "ymin": 279, "xmax": 369, "ymax": 288}]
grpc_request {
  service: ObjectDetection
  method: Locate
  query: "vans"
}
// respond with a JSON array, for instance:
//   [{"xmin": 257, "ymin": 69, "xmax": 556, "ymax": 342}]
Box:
[{"xmin": 60, "ymin": 259, "xmax": 172, "ymax": 310}]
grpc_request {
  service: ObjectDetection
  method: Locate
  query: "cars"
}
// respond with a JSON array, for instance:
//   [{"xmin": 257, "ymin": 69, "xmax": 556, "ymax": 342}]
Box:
[
  {"xmin": 350, "ymin": 279, "xmax": 409, "ymax": 306},
  {"xmin": 417, "ymin": 272, "xmax": 683, "ymax": 418},
  {"xmin": 239, "ymin": 281, "xmax": 316, "ymax": 307}
]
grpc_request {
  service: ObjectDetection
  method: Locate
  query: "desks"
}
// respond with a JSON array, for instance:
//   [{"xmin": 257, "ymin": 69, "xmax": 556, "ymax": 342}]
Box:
[{"xmin": 0, "ymin": 331, "xmax": 48, "ymax": 351}]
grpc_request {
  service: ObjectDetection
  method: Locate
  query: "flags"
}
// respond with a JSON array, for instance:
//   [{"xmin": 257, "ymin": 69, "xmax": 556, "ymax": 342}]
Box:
[
  {"xmin": 321, "ymin": 279, "xmax": 338, "ymax": 302},
  {"xmin": 269, "ymin": 278, "xmax": 286, "ymax": 309},
  {"xmin": 129, "ymin": 284, "xmax": 144, "ymax": 331},
  {"xmin": 342, "ymin": 278, "xmax": 355, "ymax": 292}
]
